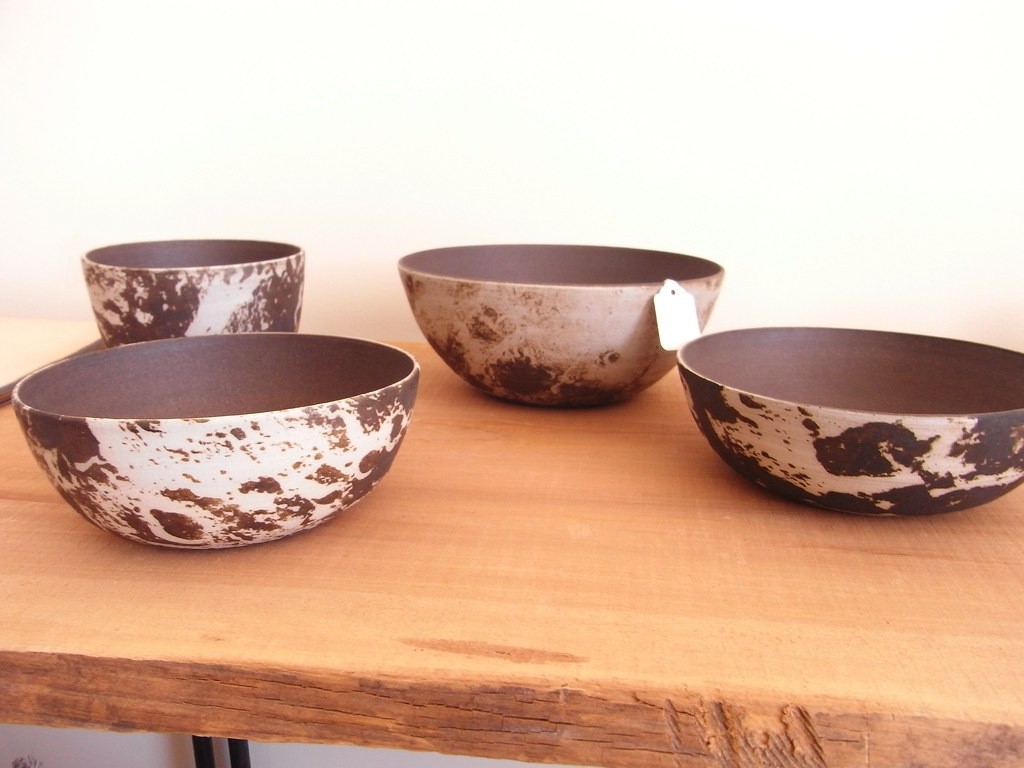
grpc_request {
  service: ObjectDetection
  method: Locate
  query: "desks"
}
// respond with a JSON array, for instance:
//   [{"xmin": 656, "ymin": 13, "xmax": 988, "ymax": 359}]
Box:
[{"xmin": 0, "ymin": 343, "xmax": 1024, "ymax": 768}]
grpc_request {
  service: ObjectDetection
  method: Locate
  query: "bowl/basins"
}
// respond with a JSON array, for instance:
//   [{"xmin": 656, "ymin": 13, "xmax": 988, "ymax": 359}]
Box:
[
  {"xmin": 676, "ymin": 327, "xmax": 1024, "ymax": 520},
  {"xmin": 398, "ymin": 244, "xmax": 724, "ymax": 404},
  {"xmin": 11, "ymin": 332, "xmax": 419, "ymax": 550},
  {"xmin": 79, "ymin": 239, "xmax": 304, "ymax": 348}
]
